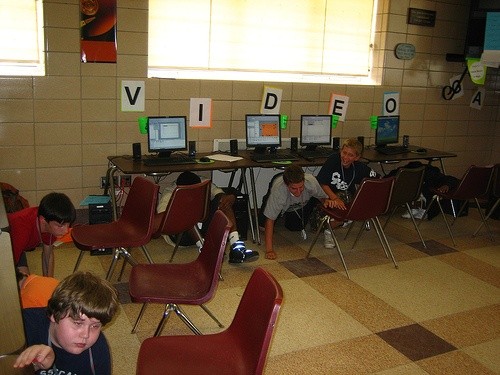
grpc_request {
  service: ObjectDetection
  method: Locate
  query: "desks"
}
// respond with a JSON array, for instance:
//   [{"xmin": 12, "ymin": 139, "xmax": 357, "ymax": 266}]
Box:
[
  {"xmin": 107, "ymin": 151, "xmax": 257, "ymax": 243},
  {"xmin": 227, "ymin": 147, "xmax": 331, "ymax": 247},
  {"xmin": 360, "ymin": 144, "xmax": 457, "ymax": 176}
]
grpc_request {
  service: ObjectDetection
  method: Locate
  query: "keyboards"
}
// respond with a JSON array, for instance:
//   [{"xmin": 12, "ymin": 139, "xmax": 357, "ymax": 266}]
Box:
[
  {"xmin": 299, "ymin": 152, "xmax": 334, "ymax": 160},
  {"xmin": 144, "ymin": 158, "xmax": 198, "ymax": 167},
  {"xmin": 252, "ymin": 155, "xmax": 299, "ymax": 162},
  {"xmin": 376, "ymin": 147, "xmax": 410, "ymax": 156}
]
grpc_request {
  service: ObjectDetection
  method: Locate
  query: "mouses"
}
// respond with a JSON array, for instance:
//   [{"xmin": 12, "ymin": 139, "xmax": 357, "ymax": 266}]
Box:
[
  {"xmin": 200, "ymin": 158, "xmax": 210, "ymax": 161},
  {"xmin": 417, "ymin": 148, "xmax": 427, "ymax": 152}
]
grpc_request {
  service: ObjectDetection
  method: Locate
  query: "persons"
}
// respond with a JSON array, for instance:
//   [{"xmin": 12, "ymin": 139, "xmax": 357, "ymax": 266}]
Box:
[
  {"xmin": 13, "ymin": 271, "xmax": 119, "ymax": 375},
  {"xmin": 157, "ymin": 172, "xmax": 259, "ymax": 264},
  {"xmin": 264, "ymin": 163, "xmax": 337, "ymax": 259},
  {"xmin": 1, "ymin": 193, "xmax": 77, "ymax": 289},
  {"xmin": 317, "ymin": 138, "xmax": 385, "ymax": 249}
]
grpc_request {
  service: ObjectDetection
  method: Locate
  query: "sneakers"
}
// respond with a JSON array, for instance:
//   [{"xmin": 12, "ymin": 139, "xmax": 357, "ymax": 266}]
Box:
[
  {"xmin": 401, "ymin": 208, "xmax": 418, "ymax": 218},
  {"xmin": 324, "ymin": 230, "xmax": 335, "ymax": 249},
  {"xmin": 197, "ymin": 239, "xmax": 204, "ymax": 253},
  {"xmin": 228, "ymin": 239, "xmax": 260, "ymax": 263},
  {"xmin": 414, "ymin": 209, "xmax": 428, "ymax": 220}
]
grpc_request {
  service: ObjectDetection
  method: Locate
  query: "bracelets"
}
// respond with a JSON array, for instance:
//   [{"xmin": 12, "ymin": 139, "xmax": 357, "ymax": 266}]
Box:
[{"xmin": 332, "ymin": 197, "xmax": 339, "ymax": 201}]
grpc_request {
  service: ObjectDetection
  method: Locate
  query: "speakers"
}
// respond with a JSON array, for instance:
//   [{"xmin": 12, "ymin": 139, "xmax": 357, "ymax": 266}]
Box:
[
  {"xmin": 331, "ymin": 137, "xmax": 340, "ymax": 151},
  {"xmin": 403, "ymin": 136, "xmax": 409, "ymax": 148},
  {"xmin": 357, "ymin": 136, "xmax": 364, "ymax": 149},
  {"xmin": 291, "ymin": 137, "xmax": 299, "ymax": 151},
  {"xmin": 133, "ymin": 142, "xmax": 141, "ymax": 159},
  {"xmin": 189, "ymin": 141, "xmax": 196, "ymax": 156},
  {"xmin": 230, "ymin": 140, "xmax": 238, "ymax": 154}
]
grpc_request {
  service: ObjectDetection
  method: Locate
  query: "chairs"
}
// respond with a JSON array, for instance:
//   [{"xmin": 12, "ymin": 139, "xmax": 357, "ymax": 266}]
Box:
[
  {"xmin": 305, "ymin": 177, "xmax": 399, "ymax": 280},
  {"xmin": 128, "ymin": 209, "xmax": 230, "ymax": 339},
  {"xmin": 71, "ymin": 177, "xmax": 156, "ymax": 282},
  {"xmin": 473, "ymin": 162, "xmax": 500, "ymax": 237},
  {"xmin": 152, "ymin": 179, "xmax": 226, "ymax": 281},
  {"xmin": 381, "ymin": 167, "xmax": 427, "ymax": 250},
  {"xmin": 136, "ymin": 268, "xmax": 285, "ymax": 375},
  {"xmin": 415, "ymin": 163, "xmax": 474, "ymax": 248},
  {"xmin": 450, "ymin": 164, "xmax": 494, "ymax": 240}
]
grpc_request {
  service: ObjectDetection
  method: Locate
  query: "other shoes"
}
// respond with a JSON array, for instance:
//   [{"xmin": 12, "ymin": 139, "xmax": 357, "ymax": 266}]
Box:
[
  {"xmin": 340, "ymin": 219, "xmax": 353, "ymax": 229},
  {"xmin": 365, "ymin": 221, "xmax": 371, "ymax": 231}
]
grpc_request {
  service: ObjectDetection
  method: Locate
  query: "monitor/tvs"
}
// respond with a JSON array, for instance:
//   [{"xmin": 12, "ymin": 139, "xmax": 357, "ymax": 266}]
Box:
[
  {"xmin": 245, "ymin": 114, "xmax": 282, "ymax": 153},
  {"xmin": 374, "ymin": 115, "xmax": 400, "ymax": 148},
  {"xmin": 147, "ymin": 116, "xmax": 188, "ymax": 157},
  {"xmin": 299, "ymin": 115, "xmax": 332, "ymax": 150}
]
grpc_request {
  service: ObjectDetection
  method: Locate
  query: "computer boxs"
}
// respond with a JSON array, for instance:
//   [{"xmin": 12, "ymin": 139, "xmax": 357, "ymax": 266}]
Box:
[{"xmin": 88, "ymin": 195, "xmax": 113, "ymax": 256}]
